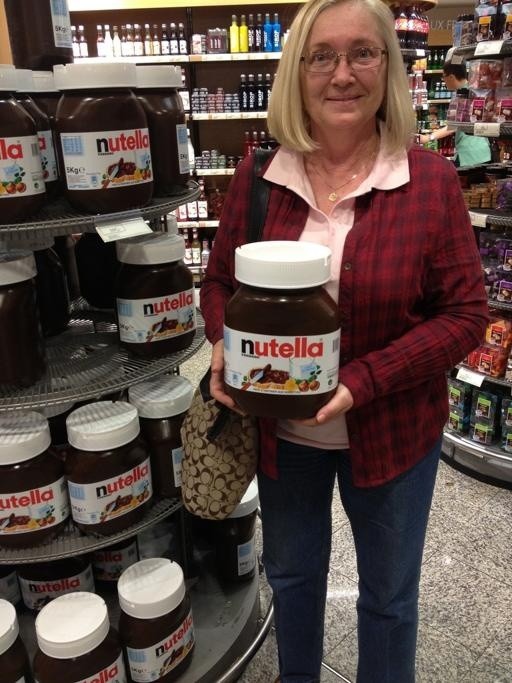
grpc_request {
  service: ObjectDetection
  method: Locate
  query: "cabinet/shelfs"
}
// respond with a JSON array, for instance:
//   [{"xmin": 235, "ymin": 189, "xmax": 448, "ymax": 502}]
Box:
[
  {"xmin": 79, "ymin": 26, "xmax": 512, "ymax": 484},
  {"xmin": 1, "ymin": 177, "xmax": 276, "ymax": 682}
]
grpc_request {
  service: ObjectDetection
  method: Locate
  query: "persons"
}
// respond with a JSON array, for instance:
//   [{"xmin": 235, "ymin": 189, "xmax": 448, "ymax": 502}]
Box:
[
  {"xmin": 199, "ymin": 0, "xmax": 489, "ymax": 681},
  {"xmin": 437, "ymin": 54, "xmax": 473, "ymax": 126}
]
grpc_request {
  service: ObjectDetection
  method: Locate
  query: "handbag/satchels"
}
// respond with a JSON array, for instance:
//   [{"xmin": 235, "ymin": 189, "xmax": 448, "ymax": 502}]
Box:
[{"xmin": 180, "ymin": 371, "xmax": 256, "ymax": 520}]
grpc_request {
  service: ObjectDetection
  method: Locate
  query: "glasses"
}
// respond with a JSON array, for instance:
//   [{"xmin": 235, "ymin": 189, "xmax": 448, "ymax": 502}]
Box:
[{"xmin": 301, "ymin": 46, "xmax": 388, "ymax": 75}]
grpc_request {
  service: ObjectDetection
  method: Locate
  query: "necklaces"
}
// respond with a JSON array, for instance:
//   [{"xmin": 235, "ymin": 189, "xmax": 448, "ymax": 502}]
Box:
[{"xmin": 299, "ymin": 135, "xmax": 379, "ymax": 205}]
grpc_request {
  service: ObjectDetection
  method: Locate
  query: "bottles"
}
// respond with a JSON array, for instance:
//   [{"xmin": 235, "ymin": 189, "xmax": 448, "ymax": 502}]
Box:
[
  {"xmin": 170, "ymin": 180, "xmax": 223, "ymax": 221},
  {"xmin": 240, "ymin": 128, "xmax": 276, "ymax": 157},
  {"xmin": 394, "ymin": 0, "xmax": 428, "ymax": 102},
  {"xmin": 175, "ymin": 227, "xmax": 215, "ymax": 265},
  {"xmin": 186, "ymin": 126, "xmax": 195, "ymax": 170},
  {"xmin": 416, "ymin": 102, "xmax": 458, "ymax": 157},
  {"xmin": 69, "ymin": 12, "xmax": 281, "ymax": 57},
  {"xmin": 176, "ymin": 68, "xmax": 192, "ymax": 113},
  {"xmin": 239, "ymin": 72, "xmax": 279, "ymax": 112},
  {"xmin": 424, "ymin": 46, "xmax": 457, "ymax": 100}
]
